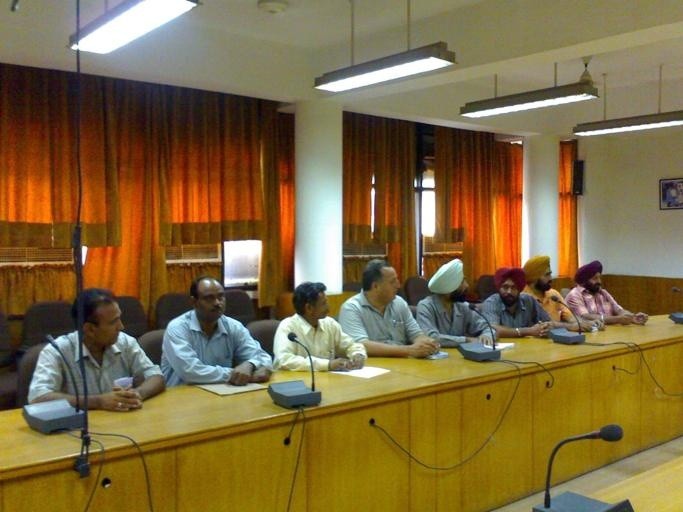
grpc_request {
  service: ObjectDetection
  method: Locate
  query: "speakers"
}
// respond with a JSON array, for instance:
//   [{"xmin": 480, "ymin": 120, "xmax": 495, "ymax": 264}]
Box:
[{"xmin": 572, "ymin": 160, "xmax": 584, "ymax": 196}]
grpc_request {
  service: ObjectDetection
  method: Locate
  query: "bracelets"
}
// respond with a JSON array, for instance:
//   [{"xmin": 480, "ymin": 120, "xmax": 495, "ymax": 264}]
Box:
[{"xmin": 515, "ymin": 328, "xmax": 521, "ymax": 337}]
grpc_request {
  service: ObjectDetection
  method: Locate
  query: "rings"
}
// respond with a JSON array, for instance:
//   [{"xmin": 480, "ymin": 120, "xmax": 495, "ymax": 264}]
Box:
[{"xmin": 118, "ymin": 402, "xmax": 122, "ymax": 407}]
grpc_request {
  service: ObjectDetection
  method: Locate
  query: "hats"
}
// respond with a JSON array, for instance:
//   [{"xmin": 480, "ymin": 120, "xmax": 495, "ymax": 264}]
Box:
[
  {"xmin": 493, "ymin": 266, "xmax": 526, "ymax": 293},
  {"xmin": 427, "ymin": 257, "xmax": 465, "ymax": 295},
  {"xmin": 574, "ymin": 260, "xmax": 603, "ymax": 285},
  {"xmin": 523, "ymin": 256, "xmax": 550, "ymax": 285}
]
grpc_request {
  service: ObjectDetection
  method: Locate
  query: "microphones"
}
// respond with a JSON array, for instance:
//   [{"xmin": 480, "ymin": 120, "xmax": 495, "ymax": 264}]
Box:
[
  {"xmin": 267, "ymin": 332, "xmax": 321, "ymax": 409},
  {"xmin": 22, "ymin": 334, "xmax": 85, "ymax": 434},
  {"xmin": 548, "ymin": 296, "xmax": 585, "ymax": 345},
  {"xmin": 457, "ymin": 303, "xmax": 500, "ymax": 362},
  {"xmin": 533, "ymin": 424, "xmax": 634, "ymax": 512},
  {"xmin": 669, "ymin": 287, "xmax": 683, "ymax": 324}
]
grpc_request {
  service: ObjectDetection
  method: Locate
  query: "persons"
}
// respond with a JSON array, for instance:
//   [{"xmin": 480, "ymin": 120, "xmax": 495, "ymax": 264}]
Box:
[
  {"xmin": 416, "ymin": 258, "xmax": 496, "ymax": 349},
  {"xmin": 273, "ymin": 282, "xmax": 368, "ymax": 372},
  {"xmin": 160, "ymin": 275, "xmax": 273, "ymax": 386},
  {"xmin": 26, "ymin": 288, "xmax": 166, "ymax": 412},
  {"xmin": 520, "ymin": 256, "xmax": 606, "ymax": 331},
  {"xmin": 564, "ymin": 260, "xmax": 649, "ymax": 326},
  {"xmin": 481, "ymin": 267, "xmax": 554, "ymax": 338},
  {"xmin": 338, "ymin": 259, "xmax": 441, "ymax": 359}
]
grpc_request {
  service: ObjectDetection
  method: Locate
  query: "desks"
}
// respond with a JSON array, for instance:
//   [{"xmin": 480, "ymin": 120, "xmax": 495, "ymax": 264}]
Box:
[{"xmin": 1, "ymin": 275, "xmax": 681, "ymax": 512}]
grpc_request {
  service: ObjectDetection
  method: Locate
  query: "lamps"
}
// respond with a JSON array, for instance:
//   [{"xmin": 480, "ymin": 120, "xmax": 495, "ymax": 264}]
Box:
[
  {"xmin": 573, "ymin": 62, "xmax": 683, "ymax": 137},
  {"xmin": 312, "ymin": 0, "xmax": 457, "ymax": 94},
  {"xmin": 66, "ymin": 0, "xmax": 201, "ymax": 56},
  {"xmin": 459, "ymin": 63, "xmax": 601, "ymax": 118}
]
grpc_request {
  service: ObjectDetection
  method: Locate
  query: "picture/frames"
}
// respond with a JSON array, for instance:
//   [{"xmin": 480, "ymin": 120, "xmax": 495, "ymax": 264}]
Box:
[{"xmin": 658, "ymin": 177, "xmax": 682, "ymax": 210}]
